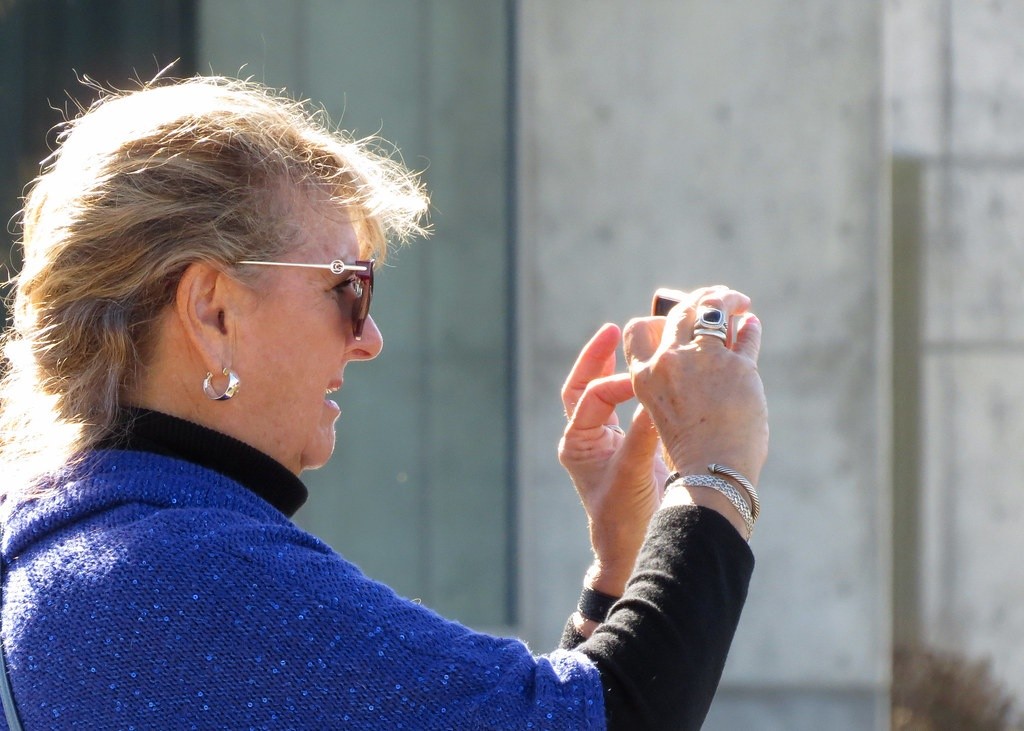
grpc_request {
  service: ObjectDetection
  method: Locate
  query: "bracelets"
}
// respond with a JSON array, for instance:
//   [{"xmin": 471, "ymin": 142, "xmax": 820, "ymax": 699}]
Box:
[
  {"xmin": 577, "ymin": 584, "xmax": 622, "ymax": 624},
  {"xmin": 662, "ymin": 463, "xmax": 760, "ymax": 542}
]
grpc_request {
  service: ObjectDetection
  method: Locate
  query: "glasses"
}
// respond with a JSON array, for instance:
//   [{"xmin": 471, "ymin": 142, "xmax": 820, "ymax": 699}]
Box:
[{"xmin": 168, "ymin": 259, "xmax": 375, "ymax": 341}]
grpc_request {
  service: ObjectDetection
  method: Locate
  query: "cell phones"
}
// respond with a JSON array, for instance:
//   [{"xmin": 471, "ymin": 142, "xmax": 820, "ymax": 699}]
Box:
[{"xmin": 651, "ymin": 289, "xmax": 732, "ymax": 350}]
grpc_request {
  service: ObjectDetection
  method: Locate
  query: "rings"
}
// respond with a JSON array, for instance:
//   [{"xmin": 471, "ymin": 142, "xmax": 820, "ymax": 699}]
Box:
[{"xmin": 693, "ymin": 307, "xmax": 728, "ymax": 346}]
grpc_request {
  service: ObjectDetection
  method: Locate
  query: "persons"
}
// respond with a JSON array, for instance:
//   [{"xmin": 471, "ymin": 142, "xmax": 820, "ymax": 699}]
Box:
[{"xmin": 0, "ymin": 54, "xmax": 772, "ymax": 731}]
